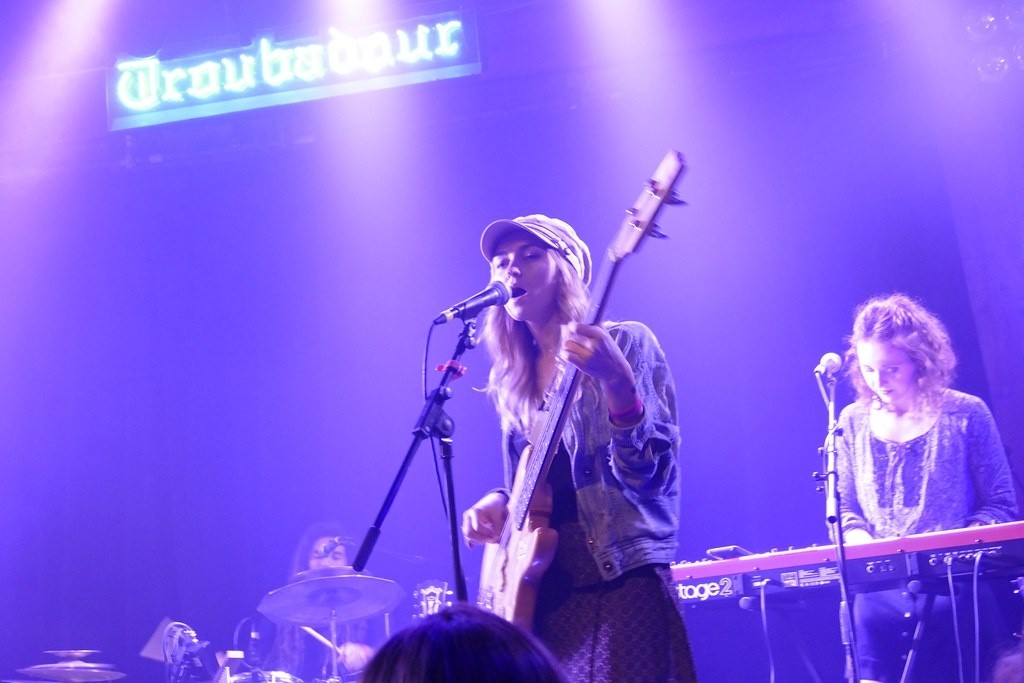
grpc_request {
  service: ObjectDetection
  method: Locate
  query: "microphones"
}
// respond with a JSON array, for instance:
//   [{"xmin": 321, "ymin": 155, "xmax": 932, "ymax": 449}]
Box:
[
  {"xmin": 813, "ymin": 352, "xmax": 843, "ymax": 375},
  {"xmin": 433, "ymin": 279, "xmax": 512, "ymax": 324}
]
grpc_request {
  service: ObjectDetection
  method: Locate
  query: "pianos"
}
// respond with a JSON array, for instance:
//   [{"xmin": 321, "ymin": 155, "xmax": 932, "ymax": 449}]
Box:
[{"xmin": 668, "ymin": 519, "xmax": 1024, "ymax": 683}]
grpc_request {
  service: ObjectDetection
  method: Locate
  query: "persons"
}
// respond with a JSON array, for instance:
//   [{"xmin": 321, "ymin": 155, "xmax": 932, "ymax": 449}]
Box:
[
  {"xmin": 836, "ymin": 291, "xmax": 1019, "ymax": 683},
  {"xmin": 290, "ymin": 517, "xmax": 375, "ymax": 672},
  {"xmin": 463, "ymin": 212, "xmax": 699, "ymax": 683},
  {"xmin": 355, "ymin": 600, "xmax": 579, "ymax": 683}
]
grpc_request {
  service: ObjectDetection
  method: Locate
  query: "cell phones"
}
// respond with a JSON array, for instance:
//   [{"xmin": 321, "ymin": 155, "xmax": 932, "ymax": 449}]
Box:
[{"xmin": 706, "ymin": 544, "xmax": 753, "ymax": 560}]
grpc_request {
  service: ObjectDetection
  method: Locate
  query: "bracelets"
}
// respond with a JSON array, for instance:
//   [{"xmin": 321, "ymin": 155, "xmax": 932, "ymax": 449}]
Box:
[{"xmin": 609, "ymin": 398, "xmax": 643, "ymax": 423}]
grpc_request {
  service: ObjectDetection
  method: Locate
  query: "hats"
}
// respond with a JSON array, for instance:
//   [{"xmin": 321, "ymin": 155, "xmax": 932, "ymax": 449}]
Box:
[{"xmin": 480, "ymin": 215, "xmax": 592, "ymax": 289}]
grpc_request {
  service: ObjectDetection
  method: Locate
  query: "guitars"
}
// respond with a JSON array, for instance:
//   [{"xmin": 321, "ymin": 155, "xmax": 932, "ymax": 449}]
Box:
[{"xmin": 476, "ymin": 148, "xmax": 690, "ymax": 636}]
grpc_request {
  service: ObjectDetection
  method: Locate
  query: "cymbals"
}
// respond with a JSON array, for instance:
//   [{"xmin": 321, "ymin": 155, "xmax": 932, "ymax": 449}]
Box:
[
  {"xmin": 256, "ymin": 567, "xmax": 408, "ymax": 629},
  {"xmin": 2, "ymin": 647, "xmax": 128, "ymax": 683}
]
